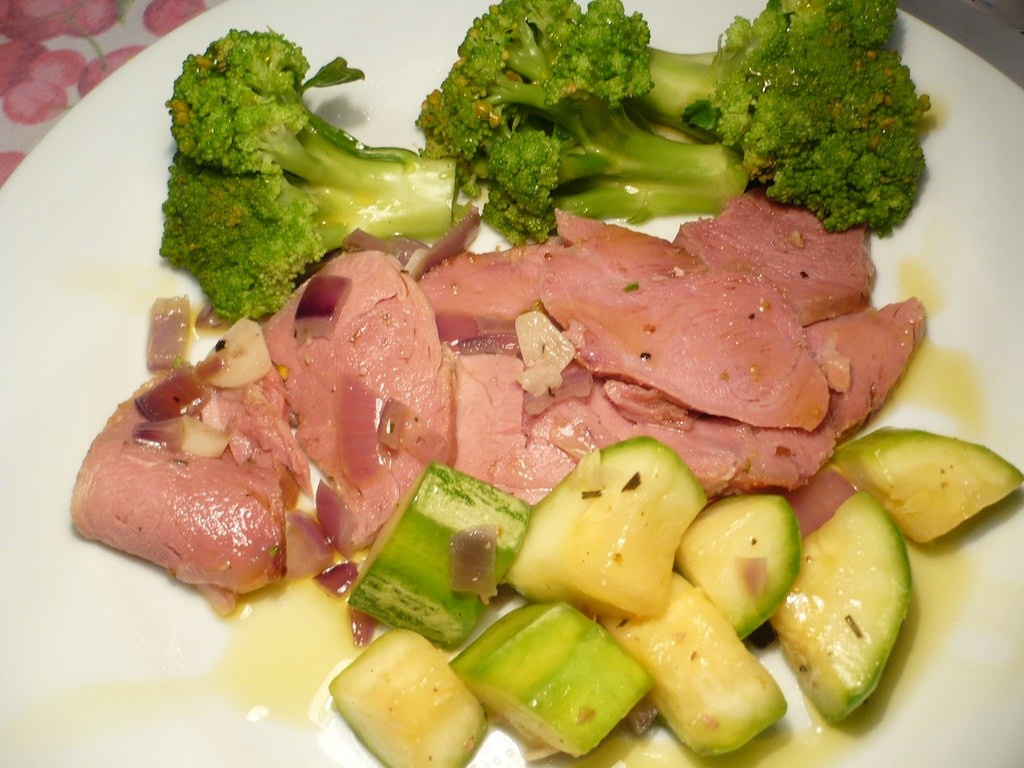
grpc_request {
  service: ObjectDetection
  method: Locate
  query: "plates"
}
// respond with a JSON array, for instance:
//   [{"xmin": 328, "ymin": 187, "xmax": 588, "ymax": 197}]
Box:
[{"xmin": 0, "ymin": 0, "xmax": 1024, "ymax": 767}]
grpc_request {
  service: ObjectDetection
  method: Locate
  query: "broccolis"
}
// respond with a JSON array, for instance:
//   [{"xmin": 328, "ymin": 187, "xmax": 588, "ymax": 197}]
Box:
[{"xmin": 157, "ymin": 0, "xmax": 933, "ymax": 322}]
